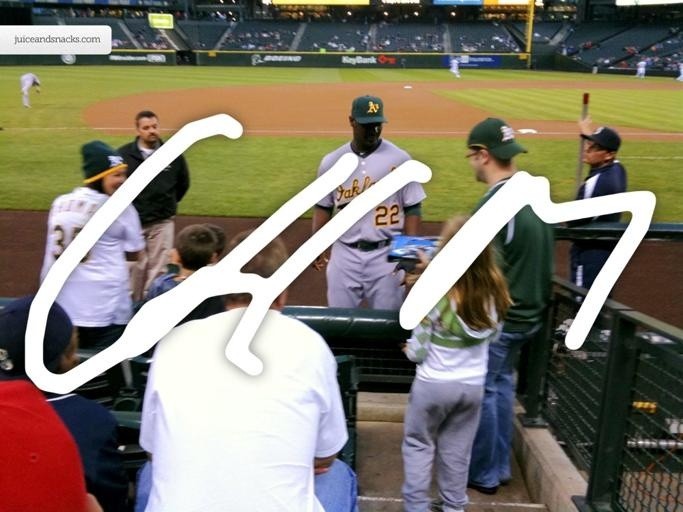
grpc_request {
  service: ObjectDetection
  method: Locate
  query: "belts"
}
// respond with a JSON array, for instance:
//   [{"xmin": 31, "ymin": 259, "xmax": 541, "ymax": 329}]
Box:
[{"xmin": 344, "ymin": 239, "xmax": 391, "ymax": 252}]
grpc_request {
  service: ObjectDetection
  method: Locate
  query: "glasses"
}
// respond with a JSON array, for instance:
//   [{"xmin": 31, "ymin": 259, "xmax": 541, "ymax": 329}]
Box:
[{"xmin": 466, "ymin": 148, "xmax": 482, "ymax": 160}]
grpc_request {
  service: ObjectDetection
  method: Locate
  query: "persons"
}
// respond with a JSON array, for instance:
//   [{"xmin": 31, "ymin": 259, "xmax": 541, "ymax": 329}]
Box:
[
  {"xmin": 0, "ymin": 297, "xmax": 127, "ymax": 512},
  {"xmin": 401, "ymin": 214, "xmax": 510, "ymax": 512},
  {"xmin": 21, "ymin": 74, "xmax": 41, "ymax": 108},
  {"xmin": 634, "ymin": 58, "xmax": 647, "ymax": 79},
  {"xmin": 203, "ymin": 223, "xmax": 227, "ymax": 260},
  {"xmin": 131, "ymin": 229, "xmax": 358, "ymax": 511},
  {"xmin": 114, "ymin": 112, "xmax": 191, "ymax": 301},
  {"xmin": 450, "ymin": 58, "xmax": 460, "ymax": 79},
  {"xmin": 567, "ymin": 115, "xmax": 628, "ymax": 302},
  {"xmin": 151, "ymin": 225, "xmax": 229, "ymax": 327},
  {"xmin": 676, "ymin": 59, "xmax": 683, "ymax": 82},
  {"xmin": 37, "ymin": 140, "xmax": 146, "ymax": 394},
  {"xmin": 464, "ymin": 117, "xmax": 552, "ymax": 493},
  {"xmin": 0, "ymin": 381, "xmax": 105, "ymax": 511},
  {"xmin": 309, "ymin": 94, "xmax": 428, "ymax": 310}
]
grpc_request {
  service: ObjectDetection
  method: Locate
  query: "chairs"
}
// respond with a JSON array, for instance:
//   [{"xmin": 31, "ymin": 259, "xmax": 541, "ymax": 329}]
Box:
[
  {"xmin": 128, "ymin": 355, "xmax": 151, "ymax": 411},
  {"xmin": 334, "ymin": 355, "xmax": 357, "ymax": 470},
  {"xmin": 70, "ymin": 350, "xmax": 119, "ymax": 407}
]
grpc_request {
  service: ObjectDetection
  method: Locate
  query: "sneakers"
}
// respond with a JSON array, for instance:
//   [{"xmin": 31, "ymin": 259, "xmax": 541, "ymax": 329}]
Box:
[
  {"xmin": 469, "ymin": 484, "xmax": 501, "ymax": 493},
  {"xmin": 500, "ymin": 479, "xmax": 511, "ymax": 484}
]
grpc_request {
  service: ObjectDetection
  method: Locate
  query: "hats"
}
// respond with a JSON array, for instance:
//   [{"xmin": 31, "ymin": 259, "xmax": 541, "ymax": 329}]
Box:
[
  {"xmin": 467, "ymin": 119, "xmax": 529, "ymax": 161},
  {"xmin": 581, "ymin": 126, "xmax": 621, "ymax": 151},
  {"xmin": 82, "ymin": 141, "xmax": 128, "ymax": 184},
  {"xmin": 0, "ymin": 296, "xmax": 75, "ymax": 374},
  {"xmin": 352, "ymin": 95, "xmax": 389, "ymax": 123}
]
